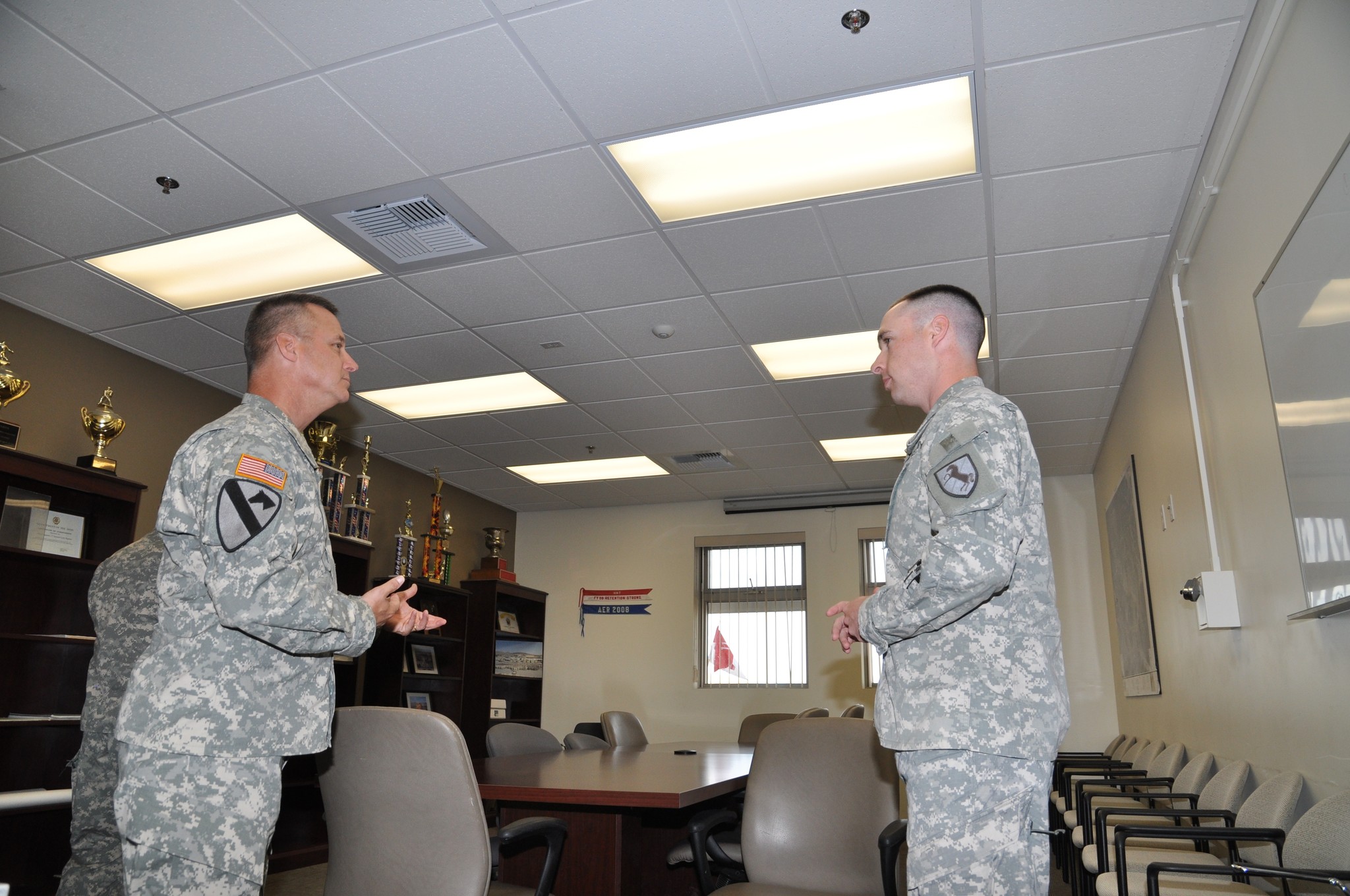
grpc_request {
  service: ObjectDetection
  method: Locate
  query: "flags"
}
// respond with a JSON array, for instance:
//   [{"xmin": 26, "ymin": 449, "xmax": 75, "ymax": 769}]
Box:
[{"xmin": 708, "ymin": 623, "xmax": 748, "ymax": 680}]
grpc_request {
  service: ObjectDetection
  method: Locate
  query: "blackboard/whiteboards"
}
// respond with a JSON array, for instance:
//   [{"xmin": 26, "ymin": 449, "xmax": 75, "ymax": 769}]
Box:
[{"xmin": 1251, "ymin": 132, "xmax": 1350, "ymax": 620}]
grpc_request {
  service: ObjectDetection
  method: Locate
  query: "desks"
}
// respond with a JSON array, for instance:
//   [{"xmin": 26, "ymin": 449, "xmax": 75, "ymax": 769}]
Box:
[
  {"xmin": 471, "ymin": 746, "xmax": 752, "ymax": 896},
  {"xmin": 589, "ymin": 740, "xmax": 753, "ymax": 886}
]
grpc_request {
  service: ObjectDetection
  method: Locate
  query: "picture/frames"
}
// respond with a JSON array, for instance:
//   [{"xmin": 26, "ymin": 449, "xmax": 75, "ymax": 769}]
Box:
[
  {"xmin": 408, "ymin": 641, "xmax": 441, "ymax": 675},
  {"xmin": 403, "ymin": 650, "xmax": 411, "ymax": 675},
  {"xmin": 401, "ymin": 688, "xmax": 433, "ymax": 712}
]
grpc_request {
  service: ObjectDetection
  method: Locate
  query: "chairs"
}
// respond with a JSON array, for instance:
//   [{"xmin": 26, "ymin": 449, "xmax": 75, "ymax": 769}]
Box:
[{"xmin": 307, "ymin": 697, "xmax": 1350, "ymax": 896}]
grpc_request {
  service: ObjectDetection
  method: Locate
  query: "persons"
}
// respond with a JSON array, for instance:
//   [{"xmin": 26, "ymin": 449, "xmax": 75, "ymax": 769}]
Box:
[
  {"xmin": 56, "ymin": 528, "xmax": 167, "ymax": 896},
  {"xmin": 116, "ymin": 295, "xmax": 446, "ymax": 896},
  {"xmin": 824, "ymin": 284, "xmax": 1069, "ymax": 896}
]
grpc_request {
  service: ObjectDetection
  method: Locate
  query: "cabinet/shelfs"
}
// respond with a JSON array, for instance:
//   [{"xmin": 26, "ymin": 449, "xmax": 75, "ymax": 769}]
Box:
[{"xmin": 0, "ymin": 445, "xmax": 551, "ymax": 896}]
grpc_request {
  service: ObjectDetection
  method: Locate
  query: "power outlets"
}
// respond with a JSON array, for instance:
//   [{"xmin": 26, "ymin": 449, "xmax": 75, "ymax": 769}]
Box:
[
  {"xmin": 1167, "ymin": 494, "xmax": 1175, "ymax": 523},
  {"xmin": 1159, "ymin": 504, "xmax": 1167, "ymax": 532}
]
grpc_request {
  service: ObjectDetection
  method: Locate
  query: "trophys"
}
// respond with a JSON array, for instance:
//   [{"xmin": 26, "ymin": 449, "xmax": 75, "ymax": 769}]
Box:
[
  {"xmin": 308, "ymin": 420, "xmax": 350, "ymax": 536},
  {"xmin": 471, "ymin": 527, "xmax": 516, "ymax": 584},
  {"xmin": 0, "ymin": 341, "xmax": 30, "ymax": 450},
  {"xmin": 76, "ymin": 387, "xmax": 126, "ymax": 476},
  {"xmin": 419, "ymin": 466, "xmax": 448, "ymax": 584},
  {"xmin": 387, "ymin": 500, "xmax": 417, "ymax": 577},
  {"xmin": 432, "ymin": 509, "xmax": 455, "ymax": 586},
  {"xmin": 344, "ymin": 435, "xmax": 376, "ymax": 545}
]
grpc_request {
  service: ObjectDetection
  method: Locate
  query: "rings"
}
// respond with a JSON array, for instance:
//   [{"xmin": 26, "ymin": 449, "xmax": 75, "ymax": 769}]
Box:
[{"xmin": 414, "ymin": 624, "xmax": 416, "ymax": 630}]
